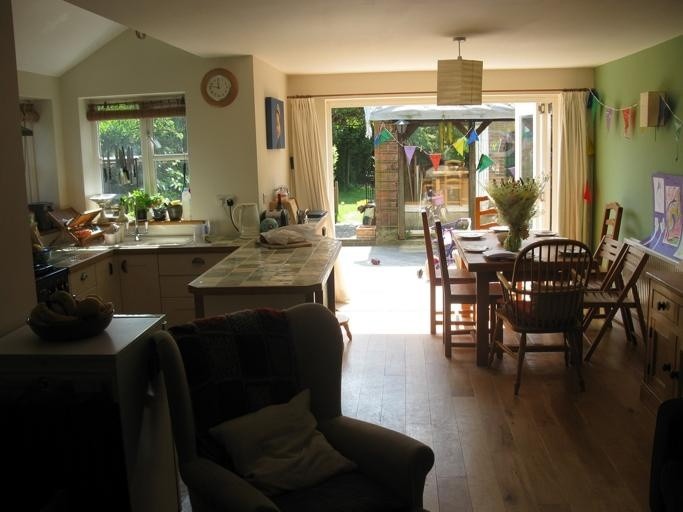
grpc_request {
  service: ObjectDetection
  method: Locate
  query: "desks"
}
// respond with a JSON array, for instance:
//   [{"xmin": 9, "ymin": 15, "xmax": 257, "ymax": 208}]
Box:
[
  {"xmin": 450, "ymin": 228, "xmax": 600, "ymax": 368},
  {"xmin": 186, "ymin": 234, "xmax": 343, "ymax": 318},
  {"xmin": 306, "ymin": 211, "xmax": 336, "ymax": 237}
]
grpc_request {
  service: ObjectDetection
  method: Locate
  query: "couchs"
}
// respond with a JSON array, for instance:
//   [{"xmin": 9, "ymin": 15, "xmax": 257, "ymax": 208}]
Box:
[{"xmin": 144, "ymin": 302, "xmax": 437, "ymax": 512}]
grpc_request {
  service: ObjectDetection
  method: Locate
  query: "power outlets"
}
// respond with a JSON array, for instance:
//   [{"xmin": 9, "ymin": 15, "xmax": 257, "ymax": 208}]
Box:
[{"xmin": 223, "ymin": 195, "xmax": 236, "ymax": 210}]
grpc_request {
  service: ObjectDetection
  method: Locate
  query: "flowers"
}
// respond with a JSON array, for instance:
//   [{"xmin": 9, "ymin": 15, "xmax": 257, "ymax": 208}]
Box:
[{"xmin": 473, "ymin": 170, "xmax": 552, "ymax": 253}]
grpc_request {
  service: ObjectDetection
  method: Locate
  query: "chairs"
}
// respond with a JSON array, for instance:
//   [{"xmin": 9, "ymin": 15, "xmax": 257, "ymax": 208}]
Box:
[
  {"xmin": 434, "ymin": 219, "xmax": 505, "ymax": 360},
  {"xmin": 473, "ymin": 196, "xmax": 498, "ymax": 230},
  {"xmin": 598, "ymin": 201, "xmax": 624, "ymax": 241},
  {"xmin": 581, "ymin": 233, "xmax": 632, "ymax": 343},
  {"xmin": 581, "ymin": 245, "xmax": 651, "ymax": 361},
  {"xmin": 420, "ymin": 208, "xmax": 477, "ymax": 336},
  {"xmin": 488, "ymin": 238, "xmax": 594, "ymax": 397}
]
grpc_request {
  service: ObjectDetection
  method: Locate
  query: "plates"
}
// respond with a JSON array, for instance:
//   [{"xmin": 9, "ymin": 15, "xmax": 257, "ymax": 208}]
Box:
[{"xmin": 457, "ymin": 226, "xmax": 590, "ymax": 263}]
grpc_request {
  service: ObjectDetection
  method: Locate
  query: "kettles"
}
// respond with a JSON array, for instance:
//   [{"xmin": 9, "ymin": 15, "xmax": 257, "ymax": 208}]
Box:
[{"xmin": 232, "ymin": 201, "xmax": 259, "ymax": 240}]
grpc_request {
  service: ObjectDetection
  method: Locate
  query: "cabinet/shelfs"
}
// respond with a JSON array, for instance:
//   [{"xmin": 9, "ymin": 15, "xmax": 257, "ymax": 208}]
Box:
[
  {"xmin": 637, "ymin": 270, "xmax": 683, "ymax": 418},
  {"xmin": 68, "ymin": 255, "xmax": 121, "ymax": 313},
  {"xmin": 114, "ymin": 255, "xmax": 162, "ymax": 314},
  {"xmin": 160, "ymin": 254, "xmax": 306, "ymax": 321},
  {"xmin": 0, "ymin": 313, "xmax": 184, "ymax": 512}
]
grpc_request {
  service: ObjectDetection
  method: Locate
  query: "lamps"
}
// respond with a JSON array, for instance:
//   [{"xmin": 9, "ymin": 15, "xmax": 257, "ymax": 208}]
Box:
[{"xmin": 435, "ymin": 37, "xmax": 485, "ymax": 107}]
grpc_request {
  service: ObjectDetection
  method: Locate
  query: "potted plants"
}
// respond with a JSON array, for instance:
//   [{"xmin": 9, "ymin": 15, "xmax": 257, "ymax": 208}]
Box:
[{"xmin": 119, "ymin": 188, "xmax": 165, "ymax": 223}]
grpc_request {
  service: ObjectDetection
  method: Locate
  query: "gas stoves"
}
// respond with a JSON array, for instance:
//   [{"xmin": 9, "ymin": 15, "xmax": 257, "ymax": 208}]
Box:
[
  {"xmin": 33, "ymin": 262, "xmax": 68, "ymax": 301},
  {"xmin": 256, "ymin": 241, "xmax": 314, "ymax": 249}
]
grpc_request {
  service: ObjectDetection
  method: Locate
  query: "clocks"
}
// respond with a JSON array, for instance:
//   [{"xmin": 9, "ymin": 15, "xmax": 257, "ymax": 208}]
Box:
[{"xmin": 198, "ymin": 67, "xmax": 238, "ymax": 108}]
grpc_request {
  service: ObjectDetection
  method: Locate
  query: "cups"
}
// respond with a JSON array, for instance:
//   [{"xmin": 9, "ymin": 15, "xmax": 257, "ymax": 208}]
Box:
[{"xmin": 193, "ymin": 224, "xmax": 209, "ymax": 242}]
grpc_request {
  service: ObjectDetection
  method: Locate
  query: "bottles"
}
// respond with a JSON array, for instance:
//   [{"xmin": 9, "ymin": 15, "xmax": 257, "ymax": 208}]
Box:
[
  {"xmin": 182, "ymin": 188, "xmax": 191, "ymax": 221},
  {"xmin": 138, "ymin": 221, "xmax": 148, "ymax": 234},
  {"xmin": 205, "ymin": 215, "xmax": 210, "ymax": 233},
  {"xmin": 128, "ymin": 221, "xmax": 136, "ymax": 234},
  {"xmin": 275, "ymin": 195, "xmax": 284, "ymax": 210}
]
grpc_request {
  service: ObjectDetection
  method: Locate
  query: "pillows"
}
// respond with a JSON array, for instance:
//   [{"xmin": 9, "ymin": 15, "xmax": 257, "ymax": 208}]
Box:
[{"xmin": 206, "ymin": 388, "xmax": 359, "ymax": 496}]
